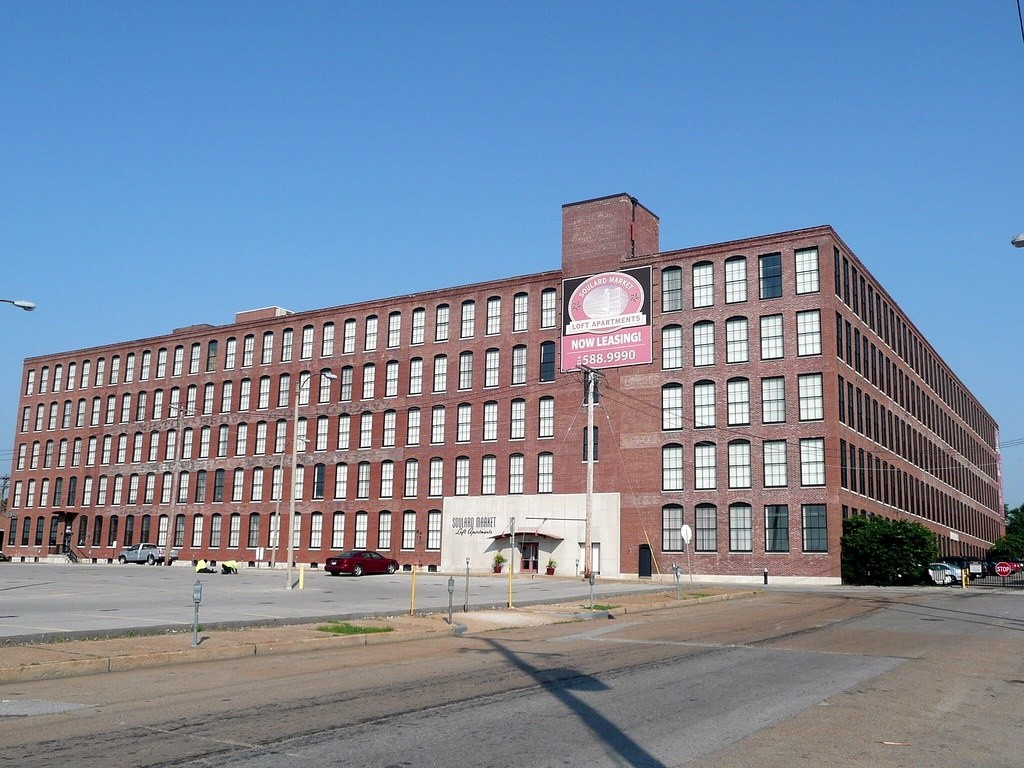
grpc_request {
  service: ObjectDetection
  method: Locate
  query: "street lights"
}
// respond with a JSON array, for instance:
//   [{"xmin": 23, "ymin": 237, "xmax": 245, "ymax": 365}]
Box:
[
  {"xmin": 270, "ymin": 438, "xmax": 310, "ymax": 569},
  {"xmin": 286, "ymin": 372, "xmax": 338, "ymax": 590}
]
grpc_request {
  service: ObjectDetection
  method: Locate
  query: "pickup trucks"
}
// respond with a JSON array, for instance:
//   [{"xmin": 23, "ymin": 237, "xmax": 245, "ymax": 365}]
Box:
[{"xmin": 118, "ymin": 543, "xmax": 179, "ymax": 566}]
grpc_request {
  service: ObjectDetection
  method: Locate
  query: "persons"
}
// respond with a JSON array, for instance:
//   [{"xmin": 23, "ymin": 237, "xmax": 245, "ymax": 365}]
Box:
[
  {"xmin": 222, "ymin": 560, "xmax": 239, "ymax": 574},
  {"xmin": 195, "ymin": 559, "xmax": 218, "ymax": 574}
]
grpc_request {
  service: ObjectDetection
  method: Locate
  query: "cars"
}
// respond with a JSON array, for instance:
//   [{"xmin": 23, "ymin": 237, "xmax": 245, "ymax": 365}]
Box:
[
  {"xmin": 324, "ymin": 550, "xmax": 399, "ymax": 577},
  {"xmin": 951, "ymin": 559, "xmax": 1024, "ymax": 582},
  {"xmin": 922, "ymin": 562, "xmax": 967, "ymax": 586}
]
